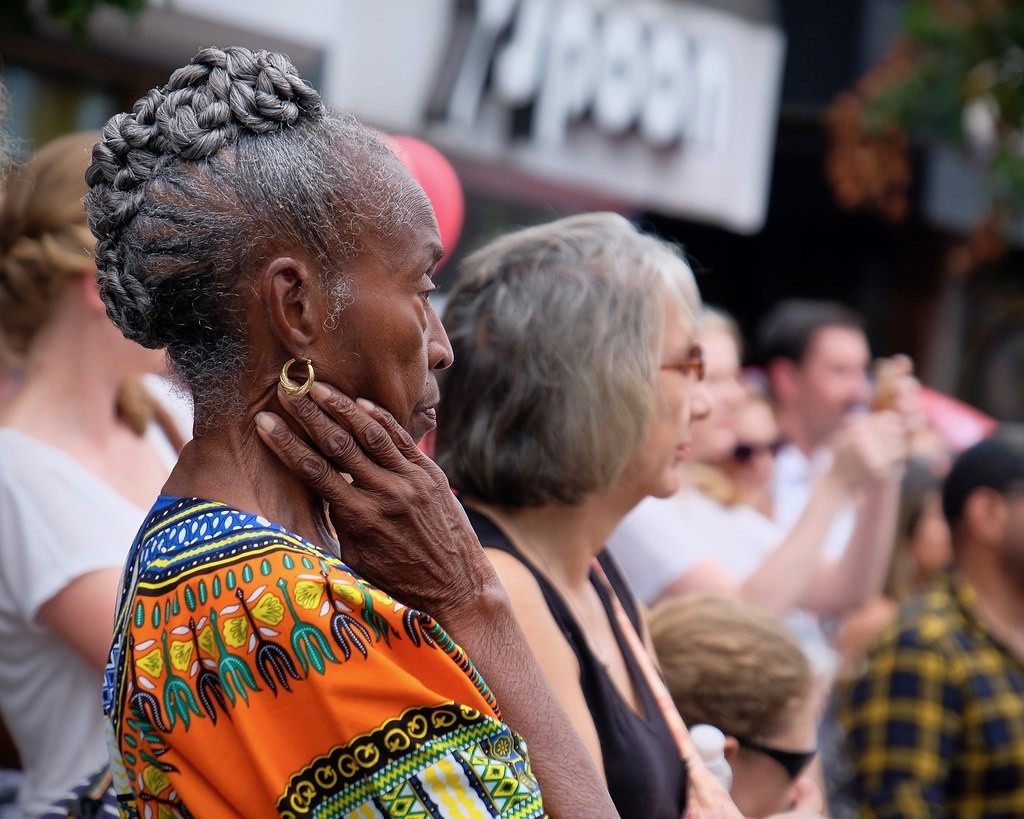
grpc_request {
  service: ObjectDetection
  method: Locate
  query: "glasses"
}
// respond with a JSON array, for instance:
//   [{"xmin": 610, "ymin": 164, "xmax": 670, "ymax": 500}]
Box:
[
  {"xmin": 685, "ymin": 713, "xmax": 818, "ymax": 782},
  {"xmin": 732, "ymin": 435, "xmax": 790, "ymax": 465},
  {"xmin": 660, "ymin": 344, "xmax": 704, "ymax": 381}
]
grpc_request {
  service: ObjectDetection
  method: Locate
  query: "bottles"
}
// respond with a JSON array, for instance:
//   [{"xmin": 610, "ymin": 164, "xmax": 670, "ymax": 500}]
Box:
[{"xmin": 688, "ymin": 724, "xmax": 733, "ymax": 794}]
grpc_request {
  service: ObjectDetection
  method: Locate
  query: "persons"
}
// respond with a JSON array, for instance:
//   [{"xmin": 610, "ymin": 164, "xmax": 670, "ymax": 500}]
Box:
[
  {"xmin": 84, "ymin": 48, "xmax": 633, "ymax": 819},
  {"xmin": 432, "ymin": 210, "xmax": 755, "ymax": 818},
  {"xmin": 0, "ymin": 63, "xmax": 1024, "ymax": 819}
]
